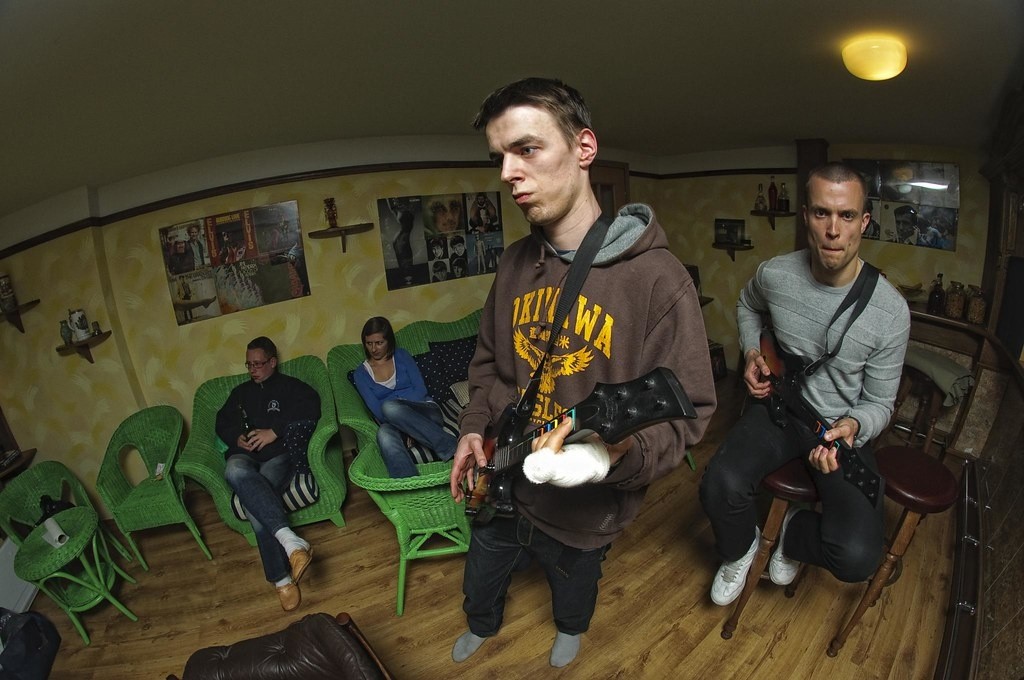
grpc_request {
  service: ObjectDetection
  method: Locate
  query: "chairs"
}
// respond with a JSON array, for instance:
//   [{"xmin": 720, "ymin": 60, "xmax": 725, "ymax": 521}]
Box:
[
  {"xmin": 347, "ymin": 442, "xmax": 488, "ymax": 618},
  {"xmin": 95, "ymin": 406, "xmax": 213, "ymax": 571},
  {"xmin": 0, "ymin": 461, "xmax": 133, "ymax": 566}
]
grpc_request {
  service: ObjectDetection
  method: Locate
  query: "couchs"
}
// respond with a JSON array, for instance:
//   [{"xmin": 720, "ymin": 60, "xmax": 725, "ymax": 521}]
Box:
[
  {"xmin": 166, "ymin": 611, "xmax": 392, "ymax": 680},
  {"xmin": 176, "ymin": 356, "xmax": 345, "ymax": 545},
  {"xmin": 328, "ymin": 307, "xmax": 496, "ymax": 453}
]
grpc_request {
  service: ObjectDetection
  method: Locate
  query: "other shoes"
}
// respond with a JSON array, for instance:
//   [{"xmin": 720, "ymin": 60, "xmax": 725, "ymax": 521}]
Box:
[
  {"xmin": 289, "ymin": 543, "xmax": 312, "ymax": 583},
  {"xmin": 277, "ymin": 575, "xmax": 302, "ymax": 612}
]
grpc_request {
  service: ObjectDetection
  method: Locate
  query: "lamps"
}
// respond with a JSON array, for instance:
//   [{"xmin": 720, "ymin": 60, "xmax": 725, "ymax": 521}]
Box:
[{"xmin": 841, "ymin": 35, "xmax": 907, "ymax": 81}]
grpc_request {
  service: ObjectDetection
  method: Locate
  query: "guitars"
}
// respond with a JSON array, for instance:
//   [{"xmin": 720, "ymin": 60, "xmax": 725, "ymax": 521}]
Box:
[
  {"xmin": 737, "ymin": 327, "xmax": 883, "ymax": 511},
  {"xmin": 463, "ymin": 366, "xmax": 699, "ymax": 529}
]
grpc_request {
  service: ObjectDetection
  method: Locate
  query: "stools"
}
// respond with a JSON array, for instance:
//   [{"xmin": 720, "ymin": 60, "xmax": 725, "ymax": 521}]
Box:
[
  {"xmin": 722, "ymin": 461, "xmax": 822, "ymax": 641},
  {"xmin": 827, "ymin": 362, "xmax": 959, "ymax": 658}
]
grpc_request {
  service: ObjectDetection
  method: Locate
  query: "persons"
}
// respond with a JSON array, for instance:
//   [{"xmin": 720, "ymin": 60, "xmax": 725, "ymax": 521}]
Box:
[
  {"xmin": 860, "ymin": 165, "xmax": 957, "ymax": 251},
  {"xmin": 699, "ymin": 163, "xmax": 912, "ymax": 605},
  {"xmin": 450, "ymin": 77, "xmax": 718, "ymax": 666},
  {"xmin": 385, "ymin": 193, "xmax": 498, "ymax": 284},
  {"xmin": 353, "ymin": 317, "xmax": 457, "ymax": 479},
  {"xmin": 165, "ymin": 224, "xmax": 246, "ymax": 322},
  {"xmin": 215, "ymin": 337, "xmax": 321, "ymax": 612}
]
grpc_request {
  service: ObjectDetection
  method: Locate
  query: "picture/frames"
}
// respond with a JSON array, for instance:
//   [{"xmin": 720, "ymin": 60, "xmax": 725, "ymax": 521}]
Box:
[{"xmin": 715, "ymin": 218, "xmax": 745, "ymax": 245}]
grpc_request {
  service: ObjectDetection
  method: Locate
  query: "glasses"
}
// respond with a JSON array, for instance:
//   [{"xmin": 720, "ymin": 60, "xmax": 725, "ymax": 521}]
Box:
[{"xmin": 244, "ymin": 358, "xmax": 272, "ymax": 368}]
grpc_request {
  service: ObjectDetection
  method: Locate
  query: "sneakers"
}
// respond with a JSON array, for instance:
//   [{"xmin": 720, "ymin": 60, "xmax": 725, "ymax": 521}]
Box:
[
  {"xmin": 711, "ymin": 523, "xmax": 760, "ymax": 607},
  {"xmin": 769, "ymin": 504, "xmax": 811, "ymax": 587}
]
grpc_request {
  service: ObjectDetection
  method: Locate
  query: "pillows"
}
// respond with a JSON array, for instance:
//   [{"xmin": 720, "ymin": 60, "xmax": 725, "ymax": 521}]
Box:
[
  {"xmin": 348, "ymin": 350, "xmax": 468, "ymax": 408},
  {"xmin": 450, "ymin": 380, "xmax": 472, "ymax": 407},
  {"xmin": 428, "ymin": 335, "xmax": 478, "ymax": 363}
]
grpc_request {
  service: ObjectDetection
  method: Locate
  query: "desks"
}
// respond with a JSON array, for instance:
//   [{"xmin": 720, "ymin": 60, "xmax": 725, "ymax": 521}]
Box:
[{"xmin": 14, "ymin": 506, "xmax": 138, "ymax": 647}]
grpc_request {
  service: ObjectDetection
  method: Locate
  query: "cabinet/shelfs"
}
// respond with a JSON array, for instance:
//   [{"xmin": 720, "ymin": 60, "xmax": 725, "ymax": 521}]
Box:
[{"xmin": 588, "ymin": 159, "xmax": 631, "ymax": 226}]
